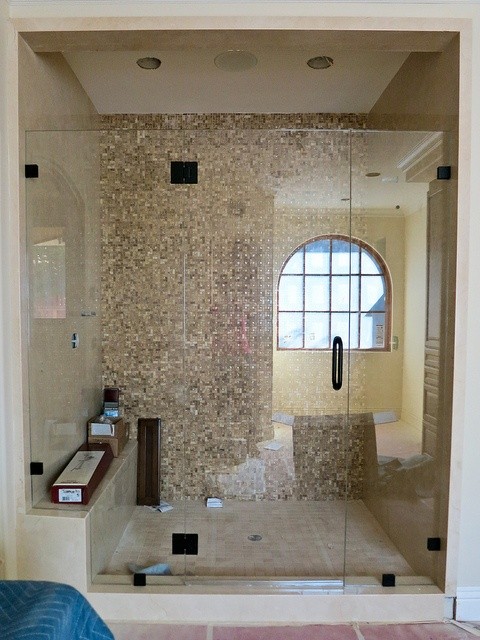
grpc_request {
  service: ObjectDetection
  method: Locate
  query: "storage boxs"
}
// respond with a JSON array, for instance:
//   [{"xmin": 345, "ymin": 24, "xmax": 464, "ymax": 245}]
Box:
[
  {"xmin": 88, "ymin": 423, "xmax": 129, "ymax": 458},
  {"xmin": 88, "ymin": 415, "xmax": 123, "ymax": 438},
  {"xmin": 272, "ymin": 410, "xmax": 399, "ymax": 490}
]
